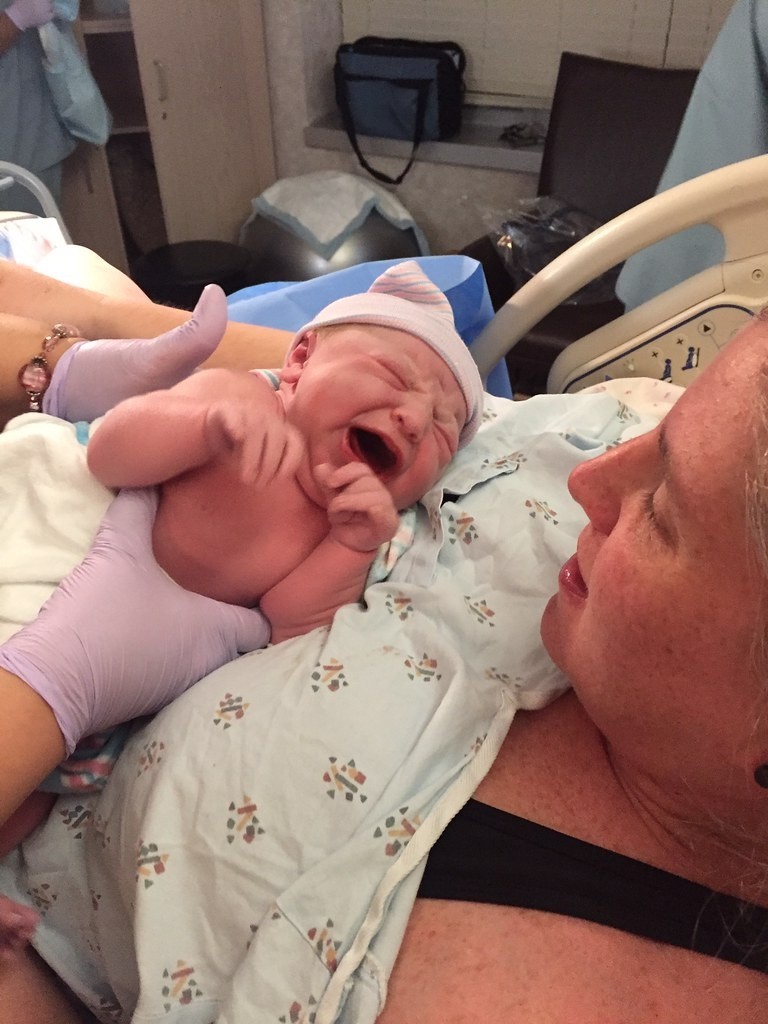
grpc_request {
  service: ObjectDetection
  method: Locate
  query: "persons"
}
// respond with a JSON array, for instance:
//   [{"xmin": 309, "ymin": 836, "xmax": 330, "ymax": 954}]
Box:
[
  {"xmin": 1, "ymin": 254, "xmax": 767, "ymax": 1024},
  {"xmin": 0, "ymin": 0, "xmax": 113, "ymax": 219},
  {"xmin": 614, "ymin": 0, "xmax": 768, "ymax": 317}
]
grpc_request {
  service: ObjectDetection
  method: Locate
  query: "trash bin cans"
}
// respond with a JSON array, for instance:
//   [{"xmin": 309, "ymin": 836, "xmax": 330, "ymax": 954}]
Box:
[{"xmin": 133, "ymin": 239, "xmax": 258, "ymax": 313}]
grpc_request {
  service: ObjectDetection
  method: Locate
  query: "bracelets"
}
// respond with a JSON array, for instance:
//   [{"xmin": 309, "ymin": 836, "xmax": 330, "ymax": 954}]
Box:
[{"xmin": 16, "ymin": 323, "xmax": 78, "ymax": 412}]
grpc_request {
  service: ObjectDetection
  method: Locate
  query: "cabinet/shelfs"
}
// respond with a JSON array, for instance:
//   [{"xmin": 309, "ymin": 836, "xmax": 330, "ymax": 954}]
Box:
[{"xmin": 51, "ymin": 0, "xmax": 278, "ymax": 280}]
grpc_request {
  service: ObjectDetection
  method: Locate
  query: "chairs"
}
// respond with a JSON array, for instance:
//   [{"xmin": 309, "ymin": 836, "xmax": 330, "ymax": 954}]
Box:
[{"xmin": 460, "ymin": 51, "xmax": 699, "ymax": 398}]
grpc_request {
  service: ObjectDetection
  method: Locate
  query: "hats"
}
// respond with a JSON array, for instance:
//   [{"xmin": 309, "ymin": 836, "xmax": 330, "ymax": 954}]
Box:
[{"xmin": 282, "ymin": 260, "xmax": 484, "ymax": 452}]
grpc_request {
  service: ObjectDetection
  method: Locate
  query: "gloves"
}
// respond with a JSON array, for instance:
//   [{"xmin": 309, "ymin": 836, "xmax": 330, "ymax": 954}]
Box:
[
  {"xmin": 0, "ymin": 484, "xmax": 272, "ymax": 761},
  {"xmin": 41, "ymin": 284, "xmax": 228, "ymax": 422}
]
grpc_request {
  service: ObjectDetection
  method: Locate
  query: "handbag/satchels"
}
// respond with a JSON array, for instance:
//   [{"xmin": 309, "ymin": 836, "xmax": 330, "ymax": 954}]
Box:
[
  {"xmin": 37, "ymin": 19, "xmax": 113, "ymax": 149},
  {"xmin": 334, "ymin": 35, "xmax": 466, "ymax": 184}
]
